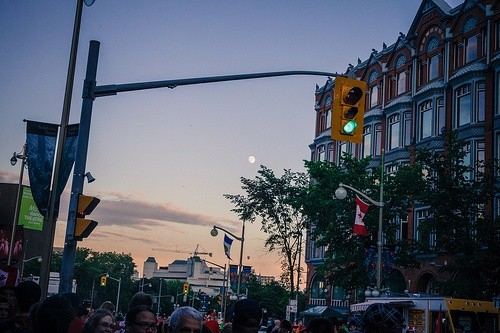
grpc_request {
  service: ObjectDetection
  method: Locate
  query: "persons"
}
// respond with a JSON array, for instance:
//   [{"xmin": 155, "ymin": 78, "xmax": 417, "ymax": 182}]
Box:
[{"xmin": 0, "ymin": 281, "xmax": 404, "ymax": 333}]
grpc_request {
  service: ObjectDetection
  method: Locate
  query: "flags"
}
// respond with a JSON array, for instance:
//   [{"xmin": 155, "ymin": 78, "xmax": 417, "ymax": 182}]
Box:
[
  {"xmin": 224, "ymin": 234, "xmax": 233, "ymax": 260},
  {"xmin": 354, "ymin": 195, "xmax": 369, "ymax": 237}
]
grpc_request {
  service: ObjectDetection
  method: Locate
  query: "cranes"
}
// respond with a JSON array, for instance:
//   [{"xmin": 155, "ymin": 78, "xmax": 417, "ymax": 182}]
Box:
[{"xmin": 152, "ymin": 243, "xmax": 212, "ymax": 259}]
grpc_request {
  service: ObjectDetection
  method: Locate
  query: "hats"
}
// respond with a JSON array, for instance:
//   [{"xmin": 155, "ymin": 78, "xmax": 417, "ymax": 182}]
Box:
[{"xmin": 233, "ymin": 299, "xmax": 262, "ymax": 327}]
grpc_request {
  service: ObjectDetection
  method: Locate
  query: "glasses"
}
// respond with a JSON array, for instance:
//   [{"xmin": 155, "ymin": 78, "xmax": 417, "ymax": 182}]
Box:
[
  {"xmin": 132, "ymin": 321, "xmax": 160, "ymax": 330},
  {"xmin": 98, "ymin": 323, "xmax": 113, "ymax": 329}
]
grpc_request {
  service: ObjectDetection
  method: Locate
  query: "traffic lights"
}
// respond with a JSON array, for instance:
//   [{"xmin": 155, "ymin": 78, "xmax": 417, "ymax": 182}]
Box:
[
  {"xmin": 101, "ymin": 277, "xmax": 106, "ymax": 286},
  {"xmin": 332, "ymin": 77, "xmax": 366, "ymax": 143},
  {"xmin": 74, "ymin": 218, "xmax": 98, "ymax": 240},
  {"xmin": 183, "ymin": 283, "xmax": 188, "ymax": 295},
  {"xmin": 78, "ymin": 194, "xmax": 101, "ymax": 216}
]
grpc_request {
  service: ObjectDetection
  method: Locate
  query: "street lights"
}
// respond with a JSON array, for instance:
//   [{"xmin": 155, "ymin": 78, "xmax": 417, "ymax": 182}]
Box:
[
  {"xmin": 335, "ymin": 182, "xmax": 384, "ymax": 289},
  {"xmin": 138, "ymin": 283, "xmax": 151, "ymax": 292},
  {"xmin": 209, "ymin": 267, "xmax": 229, "ymax": 322},
  {"xmin": 8, "ymin": 151, "xmax": 27, "ymax": 265},
  {"xmin": 211, "ymin": 226, "xmax": 244, "ymax": 295},
  {"xmin": 91, "ymin": 273, "xmax": 109, "ymax": 313},
  {"xmin": 20, "ymin": 256, "xmax": 42, "ymax": 282}
]
